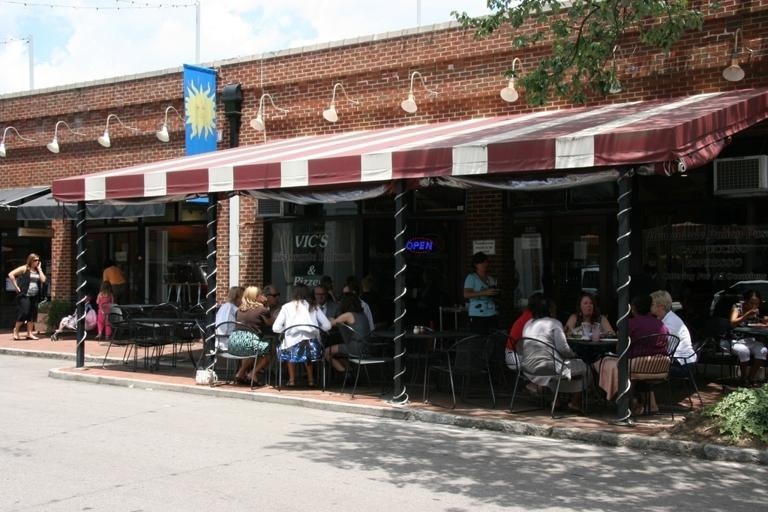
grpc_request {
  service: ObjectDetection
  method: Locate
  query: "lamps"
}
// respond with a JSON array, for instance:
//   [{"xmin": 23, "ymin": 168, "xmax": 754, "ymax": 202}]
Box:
[
  {"xmin": 401, "ymin": 71, "xmax": 436, "ymax": 114},
  {"xmin": 250, "ymin": 94, "xmax": 291, "ymax": 131},
  {"xmin": 46, "ymin": 121, "xmax": 87, "ymax": 153},
  {"xmin": 604, "ymin": 44, "xmax": 638, "ymax": 93},
  {"xmin": 722, "ymin": 28, "xmax": 746, "ymax": 82},
  {"xmin": 322, "ymin": 83, "xmax": 358, "ymax": 123},
  {"xmin": 500, "ymin": 58, "xmax": 524, "ymax": 103},
  {"xmin": 0, "ymin": 127, "xmax": 36, "ymax": 158},
  {"xmin": 156, "ymin": 106, "xmax": 185, "ymax": 142},
  {"xmin": 98, "ymin": 114, "xmax": 138, "ymax": 147}
]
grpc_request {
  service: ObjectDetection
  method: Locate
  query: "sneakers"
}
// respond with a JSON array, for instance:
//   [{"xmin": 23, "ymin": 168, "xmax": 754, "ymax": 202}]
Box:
[
  {"xmin": 13, "ymin": 327, "xmax": 19, "ymax": 340},
  {"xmin": 739, "ymin": 378, "xmax": 752, "ymax": 388},
  {"xmin": 26, "ymin": 334, "xmax": 39, "ymax": 340},
  {"xmin": 750, "ymin": 381, "xmax": 759, "ymax": 388}
]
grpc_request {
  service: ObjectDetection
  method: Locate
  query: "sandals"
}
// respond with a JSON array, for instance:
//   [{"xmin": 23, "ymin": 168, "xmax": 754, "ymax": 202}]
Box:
[
  {"xmin": 285, "ymin": 379, "xmax": 296, "ymax": 387},
  {"xmin": 306, "ymin": 384, "xmax": 320, "ymax": 388}
]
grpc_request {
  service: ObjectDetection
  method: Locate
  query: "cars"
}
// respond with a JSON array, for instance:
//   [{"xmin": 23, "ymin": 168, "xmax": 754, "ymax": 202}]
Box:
[
  {"xmin": 579, "ymin": 267, "xmax": 600, "ymax": 294},
  {"xmin": 148, "ymin": 260, "xmax": 212, "ymax": 285}
]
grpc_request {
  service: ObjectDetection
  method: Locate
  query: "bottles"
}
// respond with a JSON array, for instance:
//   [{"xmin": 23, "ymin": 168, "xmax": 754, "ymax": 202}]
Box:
[
  {"xmin": 413, "ymin": 326, "xmax": 420, "ymax": 334},
  {"xmin": 566, "ymin": 324, "xmax": 584, "ymax": 338},
  {"xmin": 420, "ymin": 326, "xmax": 425, "ymax": 334},
  {"xmin": 608, "ymin": 331, "xmax": 613, "ymax": 339}
]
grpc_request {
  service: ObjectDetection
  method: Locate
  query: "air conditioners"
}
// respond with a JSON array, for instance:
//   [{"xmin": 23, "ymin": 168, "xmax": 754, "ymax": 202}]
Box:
[
  {"xmin": 255, "ymin": 198, "xmax": 297, "ymax": 219},
  {"xmin": 711, "ymin": 154, "xmax": 767, "ymax": 198}
]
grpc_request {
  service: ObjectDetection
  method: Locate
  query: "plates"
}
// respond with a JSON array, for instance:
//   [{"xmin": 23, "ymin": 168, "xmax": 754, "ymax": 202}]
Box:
[{"xmin": 747, "ymin": 323, "xmax": 767, "ymax": 328}]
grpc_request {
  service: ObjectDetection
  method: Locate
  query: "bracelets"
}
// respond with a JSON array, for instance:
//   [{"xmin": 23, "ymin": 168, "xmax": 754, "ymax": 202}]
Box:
[{"xmin": 38, "ymin": 268, "xmax": 42, "ymax": 272}]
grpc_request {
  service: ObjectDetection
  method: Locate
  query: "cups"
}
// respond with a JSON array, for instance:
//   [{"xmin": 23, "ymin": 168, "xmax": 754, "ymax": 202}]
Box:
[
  {"xmin": 581, "ymin": 322, "xmax": 591, "ymax": 339},
  {"xmin": 592, "ymin": 324, "xmax": 601, "ymax": 342}
]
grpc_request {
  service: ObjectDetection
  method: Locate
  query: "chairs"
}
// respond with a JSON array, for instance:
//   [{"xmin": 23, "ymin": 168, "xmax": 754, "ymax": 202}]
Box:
[
  {"xmin": 104, "ymin": 299, "xmax": 209, "ymax": 373},
  {"xmin": 406, "ymin": 326, "xmax": 768, "ymax": 428},
  {"xmin": 213, "ymin": 316, "xmax": 396, "ymax": 400}
]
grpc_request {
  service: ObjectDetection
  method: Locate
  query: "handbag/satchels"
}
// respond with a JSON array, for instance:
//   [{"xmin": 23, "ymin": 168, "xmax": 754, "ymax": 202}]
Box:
[{"xmin": 195, "ymin": 368, "xmax": 218, "ymax": 385}]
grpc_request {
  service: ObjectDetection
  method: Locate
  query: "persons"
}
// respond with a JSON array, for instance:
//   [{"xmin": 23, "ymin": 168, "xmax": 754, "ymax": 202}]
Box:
[
  {"xmin": 505, "ymin": 290, "xmax": 697, "ymax": 415},
  {"xmin": 215, "ymin": 276, "xmax": 375, "ymax": 386},
  {"xmin": 720, "ymin": 290, "xmax": 768, "ymax": 388},
  {"xmin": 8, "ymin": 253, "xmax": 46, "ymax": 340},
  {"xmin": 94, "ymin": 280, "xmax": 114, "ymax": 340},
  {"xmin": 464, "ymin": 252, "xmax": 500, "ymax": 332},
  {"xmin": 102, "ymin": 262, "xmax": 129, "ymax": 305}
]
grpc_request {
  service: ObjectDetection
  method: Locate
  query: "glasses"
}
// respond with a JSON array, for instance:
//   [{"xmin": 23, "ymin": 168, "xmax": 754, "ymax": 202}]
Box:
[
  {"xmin": 266, "ymin": 293, "xmax": 280, "ymax": 297},
  {"xmin": 32, "ymin": 259, "xmax": 39, "ymax": 262}
]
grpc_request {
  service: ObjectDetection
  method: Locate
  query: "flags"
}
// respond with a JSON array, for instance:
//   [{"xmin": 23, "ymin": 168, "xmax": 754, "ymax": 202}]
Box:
[{"xmin": 183, "ymin": 63, "xmax": 217, "ymax": 156}]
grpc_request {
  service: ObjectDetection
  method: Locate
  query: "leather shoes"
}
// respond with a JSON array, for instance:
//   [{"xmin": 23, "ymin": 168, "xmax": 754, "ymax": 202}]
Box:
[
  {"xmin": 235, "ymin": 373, "xmax": 251, "ymax": 384},
  {"xmin": 568, "ymin": 403, "xmax": 584, "ymax": 416},
  {"xmin": 246, "ymin": 372, "xmax": 263, "ymax": 386}
]
